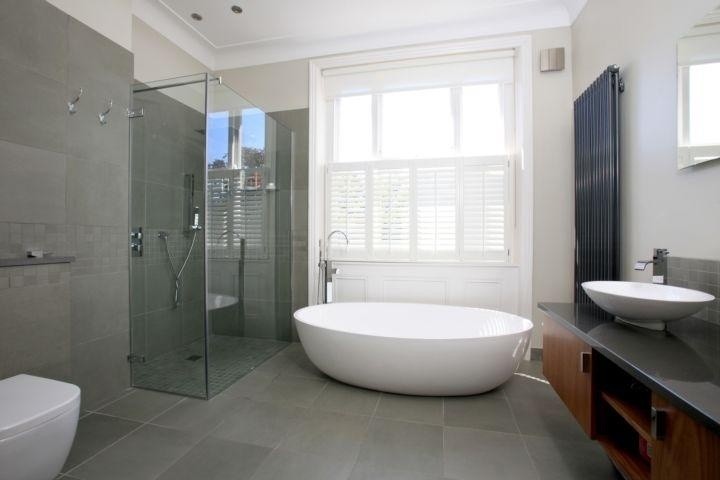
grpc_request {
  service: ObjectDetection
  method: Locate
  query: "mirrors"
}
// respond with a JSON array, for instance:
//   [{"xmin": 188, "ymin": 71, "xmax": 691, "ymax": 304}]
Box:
[{"xmin": 674, "ymin": 4, "xmax": 720, "ymax": 170}]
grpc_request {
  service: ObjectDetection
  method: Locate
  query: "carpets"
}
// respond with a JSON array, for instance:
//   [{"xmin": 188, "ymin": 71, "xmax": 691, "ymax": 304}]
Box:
[{"xmin": 133, "ymin": 333, "xmax": 292, "ymax": 402}]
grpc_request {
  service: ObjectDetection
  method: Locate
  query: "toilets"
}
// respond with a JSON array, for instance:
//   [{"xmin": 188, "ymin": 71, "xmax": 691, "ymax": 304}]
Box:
[{"xmin": 0, "ymin": 374, "xmax": 82, "ymax": 480}]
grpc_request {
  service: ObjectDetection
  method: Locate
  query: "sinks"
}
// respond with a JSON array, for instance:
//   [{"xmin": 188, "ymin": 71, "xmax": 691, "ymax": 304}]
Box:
[
  {"xmin": 581, "ymin": 280, "xmax": 716, "ymax": 333},
  {"xmin": 293, "ymin": 304, "xmax": 533, "ymax": 396}
]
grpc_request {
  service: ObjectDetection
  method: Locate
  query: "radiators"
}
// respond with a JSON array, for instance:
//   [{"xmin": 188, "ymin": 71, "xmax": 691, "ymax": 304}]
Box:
[{"xmin": 572, "ymin": 64, "xmax": 624, "ymax": 303}]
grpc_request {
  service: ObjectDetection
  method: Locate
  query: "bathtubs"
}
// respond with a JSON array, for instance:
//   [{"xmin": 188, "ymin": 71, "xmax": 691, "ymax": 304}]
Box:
[{"xmin": 209, "ymin": 294, "xmax": 238, "ymax": 311}]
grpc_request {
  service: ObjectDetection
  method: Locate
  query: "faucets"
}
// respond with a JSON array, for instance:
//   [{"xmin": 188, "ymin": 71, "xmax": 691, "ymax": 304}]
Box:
[
  {"xmin": 216, "ymin": 231, "xmax": 243, "ymax": 251},
  {"xmin": 325, "ymin": 231, "xmax": 351, "ymax": 262},
  {"xmin": 634, "ymin": 249, "xmax": 669, "ymax": 284}
]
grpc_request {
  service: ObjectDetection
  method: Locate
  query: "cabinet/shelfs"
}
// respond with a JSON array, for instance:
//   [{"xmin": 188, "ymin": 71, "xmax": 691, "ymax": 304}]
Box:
[{"xmin": 536, "ymin": 302, "xmax": 720, "ymax": 479}]
